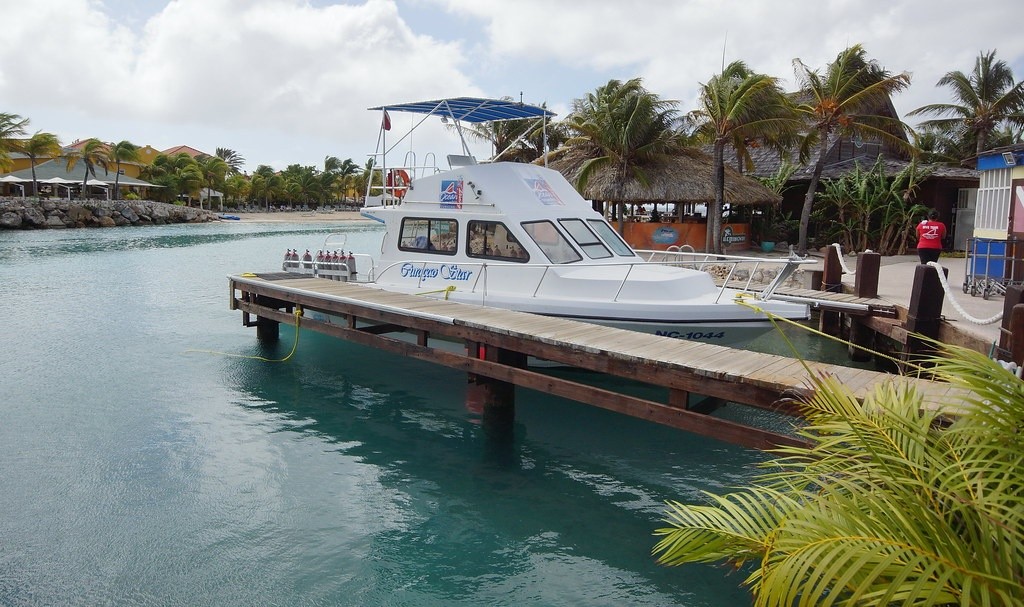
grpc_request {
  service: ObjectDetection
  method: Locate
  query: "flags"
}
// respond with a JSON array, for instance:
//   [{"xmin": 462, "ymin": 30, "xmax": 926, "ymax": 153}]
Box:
[{"xmin": 381, "ymin": 110, "xmax": 391, "ymax": 130}]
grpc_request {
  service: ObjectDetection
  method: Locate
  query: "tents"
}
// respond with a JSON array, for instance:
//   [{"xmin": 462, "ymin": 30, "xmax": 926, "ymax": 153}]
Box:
[
  {"xmin": 0, "ymin": 156, "xmax": 167, "ymax": 200},
  {"xmin": 365, "ymin": 96, "xmax": 558, "ymax": 209},
  {"xmin": 176, "ymin": 188, "xmax": 224, "ymax": 211}
]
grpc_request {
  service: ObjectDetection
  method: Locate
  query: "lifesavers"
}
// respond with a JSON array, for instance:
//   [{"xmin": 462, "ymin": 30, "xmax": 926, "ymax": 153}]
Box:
[{"xmin": 387, "ymin": 169, "xmax": 411, "ymax": 197}]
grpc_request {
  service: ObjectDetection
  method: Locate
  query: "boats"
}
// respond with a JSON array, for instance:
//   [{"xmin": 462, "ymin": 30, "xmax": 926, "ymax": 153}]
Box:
[{"xmin": 310, "ymin": 96, "xmax": 814, "ymax": 368}]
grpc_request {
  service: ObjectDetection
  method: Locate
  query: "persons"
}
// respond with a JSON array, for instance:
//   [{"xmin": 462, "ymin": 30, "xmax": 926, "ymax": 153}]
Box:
[{"xmin": 916, "ymin": 208, "xmax": 946, "ymax": 265}]
